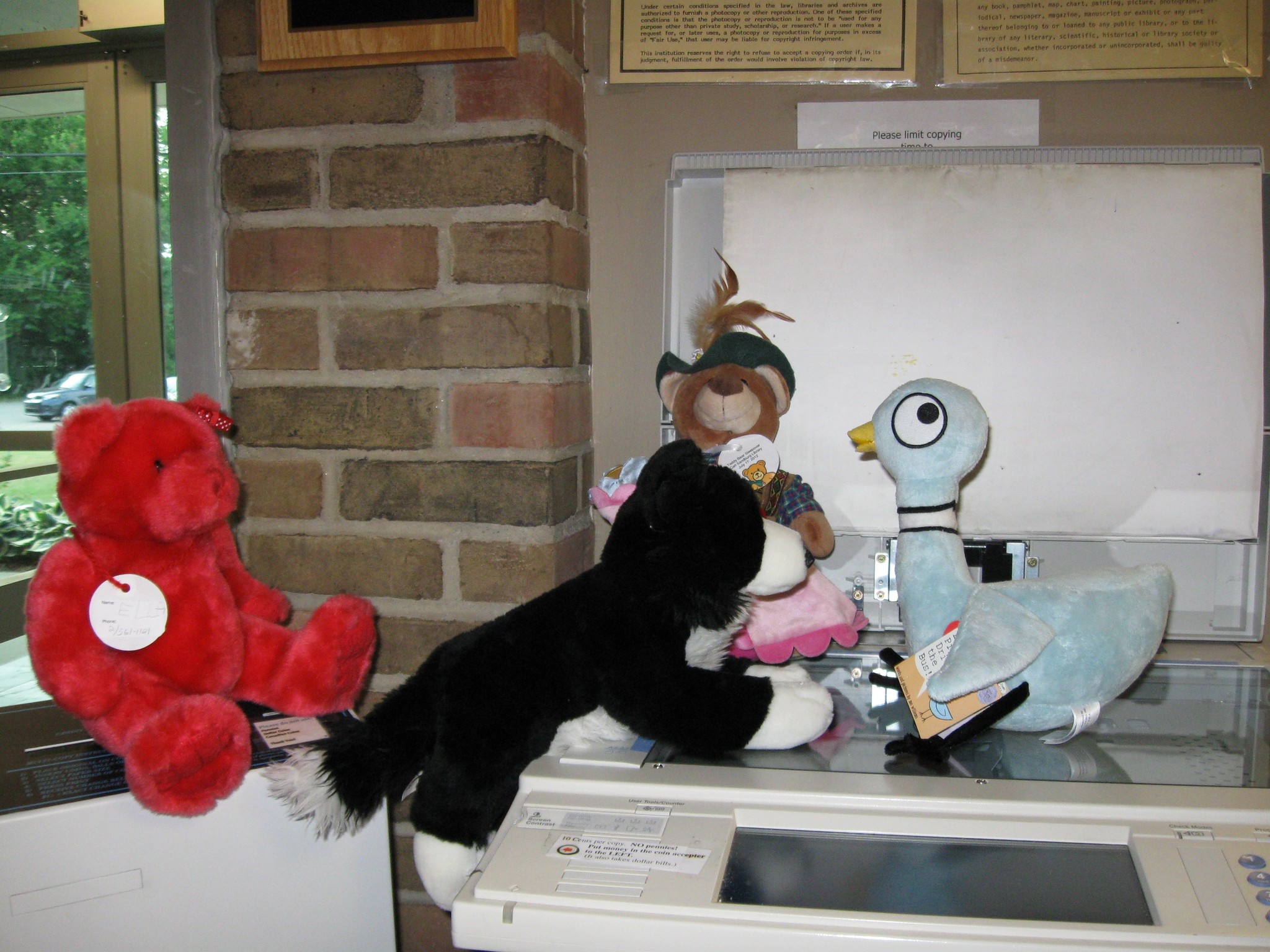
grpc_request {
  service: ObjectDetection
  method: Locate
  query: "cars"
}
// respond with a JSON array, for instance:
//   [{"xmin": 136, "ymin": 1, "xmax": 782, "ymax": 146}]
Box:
[
  {"xmin": 23, "ymin": 369, "xmax": 95, "ymax": 421},
  {"xmin": 165, "ymin": 376, "xmax": 177, "ymax": 401}
]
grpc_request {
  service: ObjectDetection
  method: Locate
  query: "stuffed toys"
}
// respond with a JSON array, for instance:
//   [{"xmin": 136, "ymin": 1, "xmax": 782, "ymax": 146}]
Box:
[
  {"xmin": 848, "ymin": 377, "xmax": 1172, "ymax": 761},
  {"xmin": 24, "ymin": 392, "xmax": 376, "ymax": 816},
  {"xmin": 268, "ymin": 437, "xmax": 833, "ymax": 911},
  {"xmin": 590, "ymin": 247, "xmax": 870, "ymax": 664}
]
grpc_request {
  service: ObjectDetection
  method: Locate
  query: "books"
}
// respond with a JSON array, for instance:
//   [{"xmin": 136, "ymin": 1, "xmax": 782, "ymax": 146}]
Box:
[{"xmin": 893, "ymin": 628, "xmax": 1009, "ymax": 739}]
